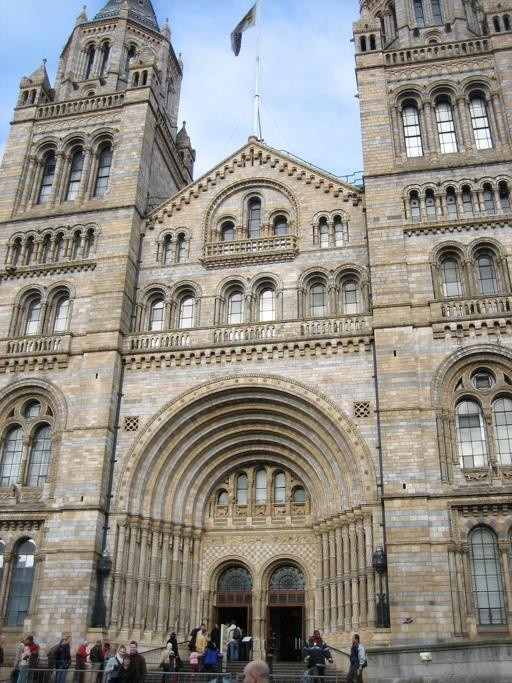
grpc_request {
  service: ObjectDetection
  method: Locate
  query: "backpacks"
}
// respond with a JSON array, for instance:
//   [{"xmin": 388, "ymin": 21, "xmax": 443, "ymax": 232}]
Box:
[
  {"xmin": 234, "ymin": 626, "xmax": 240, "ymax": 640},
  {"xmin": 47, "ymin": 644, "xmax": 62, "ymax": 659}
]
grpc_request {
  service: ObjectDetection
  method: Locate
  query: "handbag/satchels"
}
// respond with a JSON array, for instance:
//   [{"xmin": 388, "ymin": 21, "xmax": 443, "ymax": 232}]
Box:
[
  {"xmin": 175, "ymin": 656, "xmax": 183, "ymax": 668},
  {"xmin": 159, "ymin": 662, "xmax": 163, "ymax": 668}
]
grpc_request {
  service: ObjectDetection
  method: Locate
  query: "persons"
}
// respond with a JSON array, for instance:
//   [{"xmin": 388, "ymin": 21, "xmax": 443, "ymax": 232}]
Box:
[
  {"xmin": 188, "ymin": 623, "xmax": 220, "ymax": 672},
  {"xmin": 14, "ymin": 636, "xmax": 40, "ymax": 683},
  {"xmin": 46, "ymin": 636, "xmax": 146, "ymax": 683},
  {"xmin": 243, "ymin": 660, "xmax": 270, "ymax": 683},
  {"xmin": 159, "ymin": 632, "xmax": 183, "ymax": 683},
  {"xmin": 303, "ymin": 630, "xmax": 333, "ymax": 683},
  {"xmin": 226, "ymin": 620, "xmax": 242, "ymax": 662},
  {"xmin": 347, "ymin": 634, "xmax": 368, "ymax": 683},
  {"xmin": 0, "ymin": 634, "xmax": 5, "ymax": 667}
]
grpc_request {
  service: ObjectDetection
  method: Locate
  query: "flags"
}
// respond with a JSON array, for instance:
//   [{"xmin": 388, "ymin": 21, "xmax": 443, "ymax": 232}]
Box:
[{"xmin": 230, "ymin": 2, "xmax": 256, "ymax": 55}]
